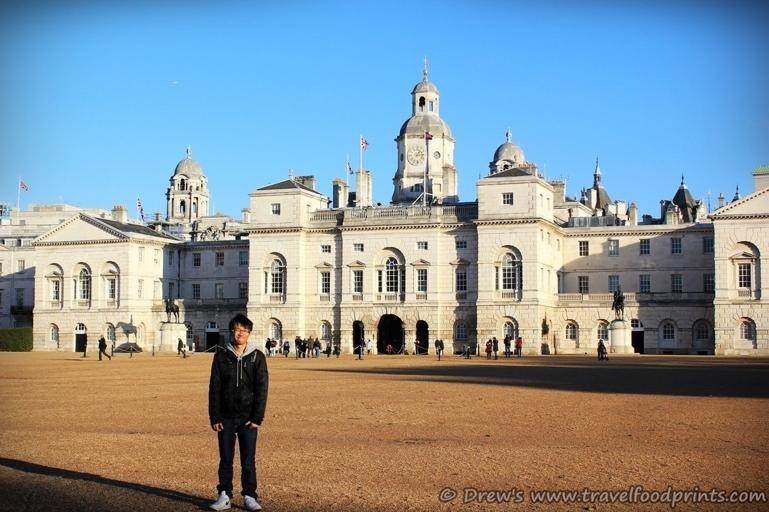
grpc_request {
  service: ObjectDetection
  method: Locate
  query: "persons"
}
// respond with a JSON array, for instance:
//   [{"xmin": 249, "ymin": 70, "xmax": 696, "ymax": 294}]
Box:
[
  {"xmin": 207, "ymin": 311, "xmax": 268, "ymax": 512},
  {"xmin": 96, "ymin": 334, "xmax": 112, "ymax": 362},
  {"xmin": 610, "ymin": 286, "xmax": 625, "ymax": 310},
  {"xmin": 176, "ymin": 337, "xmax": 184, "ymax": 357},
  {"xmin": 597, "ymin": 339, "xmax": 604, "ymax": 360},
  {"xmin": 358, "ymin": 337, "xmax": 445, "ymax": 355},
  {"xmin": 492, "ymin": 336, "xmax": 498, "ymax": 360},
  {"xmin": 515, "ymin": 336, "xmax": 522, "ymax": 358},
  {"xmin": 503, "ymin": 334, "xmax": 512, "ymax": 358},
  {"xmin": 265, "ymin": 336, "xmax": 340, "ymax": 359},
  {"xmin": 485, "ymin": 339, "xmax": 492, "ymax": 360},
  {"xmin": 164, "ymin": 295, "xmax": 176, "ymax": 312}
]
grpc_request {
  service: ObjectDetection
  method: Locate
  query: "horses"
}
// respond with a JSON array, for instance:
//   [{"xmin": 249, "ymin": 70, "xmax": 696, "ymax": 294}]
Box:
[
  {"xmin": 614, "ymin": 295, "xmax": 625, "ymax": 321},
  {"xmin": 164, "ymin": 298, "xmax": 180, "ymax": 323}
]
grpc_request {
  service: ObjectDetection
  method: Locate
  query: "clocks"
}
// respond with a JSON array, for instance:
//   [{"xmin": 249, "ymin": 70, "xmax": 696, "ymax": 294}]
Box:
[{"xmin": 407, "ymin": 146, "xmax": 424, "ymax": 166}]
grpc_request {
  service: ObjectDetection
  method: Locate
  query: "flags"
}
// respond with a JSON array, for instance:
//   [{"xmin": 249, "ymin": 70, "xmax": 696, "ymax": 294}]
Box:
[
  {"xmin": 347, "ymin": 162, "xmax": 354, "ymax": 175},
  {"xmin": 141, "ymin": 208, "xmax": 144, "ymax": 220},
  {"xmin": 361, "ymin": 137, "xmax": 369, "ymax": 151},
  {"xmin": 425, "ymin": 132, "xmax": 433, "ymax": 141},
  {"xmin": 19, "ymin": 180, "xmax": 29, "ymax": 192},
  {"xmin": 138, "ymin": 197, "xmax": 141, "ymax": 209}
]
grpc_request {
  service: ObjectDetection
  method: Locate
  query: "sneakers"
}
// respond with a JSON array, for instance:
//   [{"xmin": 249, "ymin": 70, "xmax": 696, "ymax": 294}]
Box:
[
  {"xmin": 240, "ymin": 495, "xmax": 263, "ymax": 512},
  {"xmin": 208, "ymin": 490, "xmax": 231, "ymax": 510}
]
